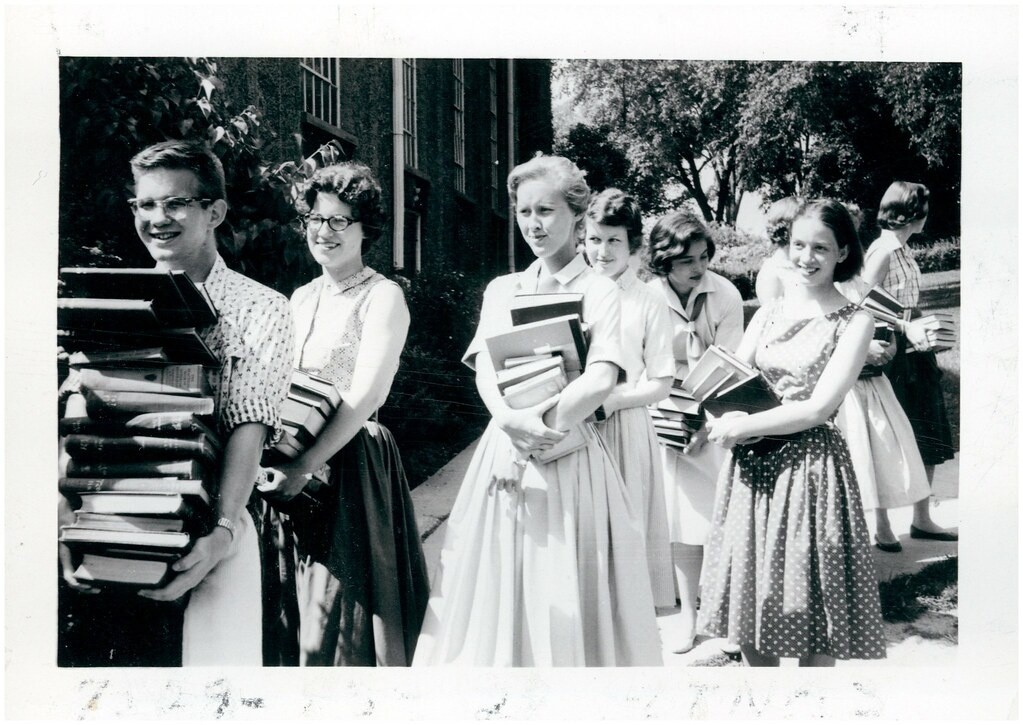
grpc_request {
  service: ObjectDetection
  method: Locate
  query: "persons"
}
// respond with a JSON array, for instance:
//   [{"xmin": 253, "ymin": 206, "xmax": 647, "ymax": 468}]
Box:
[
  {"xmin": 58, "ymin": 141, "xmax": 295, "ymax": 667},
  {"xmin": 646, "ymin": 213, "xmax": 744, "ymax": 654},
  {"xmin": 578, "ymin": 188, "xmax": 677, "ymax": 608},
  {"xmin": 755, "ymin": 195, "xmax": 931, "ymax": 510},
  {"xmin": 256, "ymin": 163, "xmax": 430, "ymax": 667},
  {"xmin": 410, "ymin": 156, "xmax": 665, "ymax": 668},
  {"xmin": 859, "ymin": 182, "xmax": 958, "ymax": 553},
  {"xmin": 697, "ymin": 199, "xmax": 887, "ymax": 669}
]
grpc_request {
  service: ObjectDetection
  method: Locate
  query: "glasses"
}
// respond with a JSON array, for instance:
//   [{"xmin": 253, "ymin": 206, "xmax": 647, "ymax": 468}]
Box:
[
  {"xmin": 128, "ymin": 197, "xmax": 213, "ymax": 220},
  {"xmin": 301, "ymin": 213, "xmax": 359, "ymax": 231}
]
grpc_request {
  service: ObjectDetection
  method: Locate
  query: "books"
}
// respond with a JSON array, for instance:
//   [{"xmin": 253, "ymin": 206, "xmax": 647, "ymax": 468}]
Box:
[
  {"xmin": 646, "ymin": 378, "xmax": 708, "ymax": 451},
  {"xmin": 859, "ymin": 282, "xmax": 904, "ymax": 378},
  {"xmin": 680, "ymin": 343, "xmax": 802, "ymax": 459},
  {"xmin": 58, "ymin": 270, "xmax": 224, "ymax": 609},
  {"xmin": 485, "ymin": 293, "xmax": 606, "ymax": 467},
  {"xmin": 905, "ymin": 314, "xmax": 956, "ymax": 354},
  {"xmin": 253, "ymin": 370, "xmax": 343, "ymax": 521}
]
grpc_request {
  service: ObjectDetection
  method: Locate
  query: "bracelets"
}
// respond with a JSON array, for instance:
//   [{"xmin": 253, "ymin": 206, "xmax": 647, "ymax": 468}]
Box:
[{"xmin": 218, "ymin": 518, "xmax": 238, "ymax": 543}]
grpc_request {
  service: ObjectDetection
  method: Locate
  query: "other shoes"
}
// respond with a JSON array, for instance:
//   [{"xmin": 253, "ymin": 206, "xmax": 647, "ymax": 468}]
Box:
[
  {"xmin": 910, "ymin": 523, "xmax": 958, "ymax": 541},
  {"xmin": 874, "ymin": 534, "xmax": 902, "ymax": 552},
  {"xmin": 669, "ymin": 625, "xmax": 697, "ymax": 653}
]
